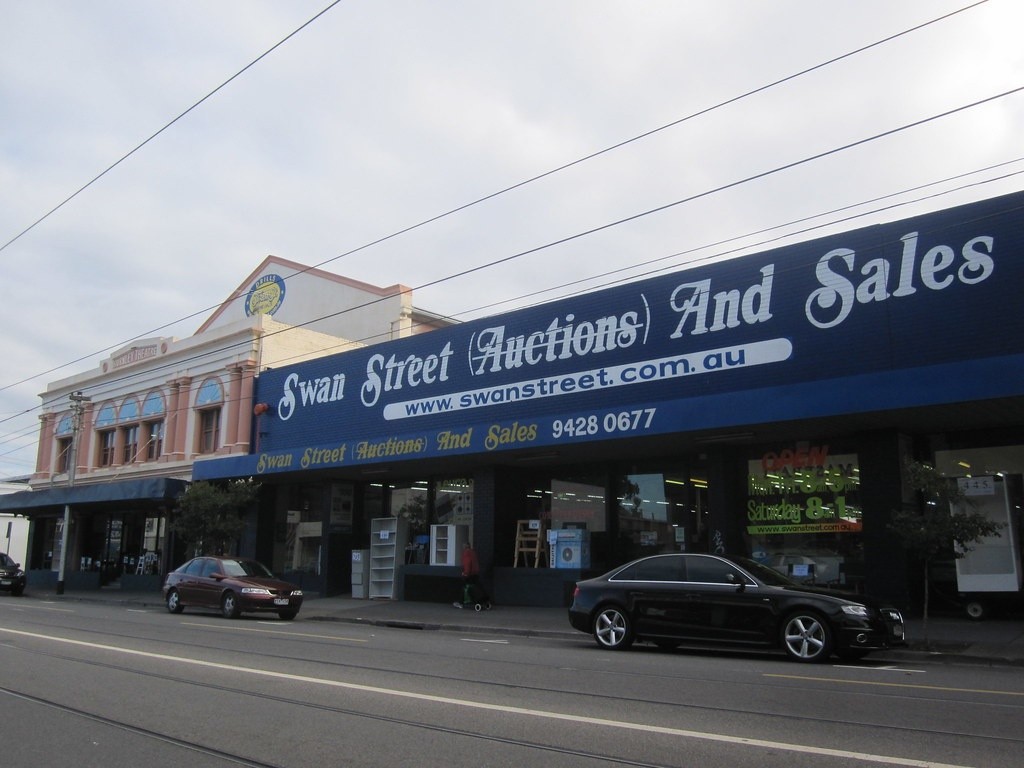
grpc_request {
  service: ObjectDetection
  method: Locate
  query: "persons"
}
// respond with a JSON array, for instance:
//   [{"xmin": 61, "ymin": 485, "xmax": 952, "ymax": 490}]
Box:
[{"xmin": 453, "ymin": 542, "xmax": 491, "ymax": 611}]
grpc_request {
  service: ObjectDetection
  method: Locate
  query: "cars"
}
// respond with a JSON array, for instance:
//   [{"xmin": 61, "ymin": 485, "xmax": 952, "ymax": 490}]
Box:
[
  {"xmin": 162, "ymin": 555, "xmax": 303, "ymax": 620},
  {"xmin": 0, "ymin": 552, "xmax": 27, "ymax": 598},
  {"xmin": 567, "ymin": 550, "xmax": 906, "ymax": 662}
]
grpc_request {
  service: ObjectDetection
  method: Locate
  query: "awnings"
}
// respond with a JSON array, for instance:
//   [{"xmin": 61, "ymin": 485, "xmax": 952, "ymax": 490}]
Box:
[{"xmin": 0, "ymin": 478, "xmax": 191, "ymax": 517}]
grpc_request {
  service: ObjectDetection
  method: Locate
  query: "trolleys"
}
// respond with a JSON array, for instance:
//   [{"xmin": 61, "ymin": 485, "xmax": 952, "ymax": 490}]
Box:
[{"xmin": 461, "ymin": 576, "xmax": 492, "ymax": 611}]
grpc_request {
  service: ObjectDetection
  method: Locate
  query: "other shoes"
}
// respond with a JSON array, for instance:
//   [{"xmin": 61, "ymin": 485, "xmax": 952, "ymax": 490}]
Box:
[{"xmin": 452, "ymin": 601, "xmax": 463, "ymax": 608}]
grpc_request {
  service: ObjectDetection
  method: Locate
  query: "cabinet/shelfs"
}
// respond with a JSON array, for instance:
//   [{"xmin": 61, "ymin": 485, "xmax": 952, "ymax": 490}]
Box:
[
  {"xmin": 351, "ymin": 549, "xmax": 368, "ymax": 598},
  {"xmin": 368, "ymin": 518, "xmax": 407, "ymax": 601},
  {"xmin": 429, "ymin": 524, "xmax": 469, "ymax": 566}
]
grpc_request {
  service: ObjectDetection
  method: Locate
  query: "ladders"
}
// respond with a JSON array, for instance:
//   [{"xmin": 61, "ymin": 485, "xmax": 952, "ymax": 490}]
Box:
[{"xmin": 513, "ymin": 519, "xmax": 550, "ymax": 569}]
